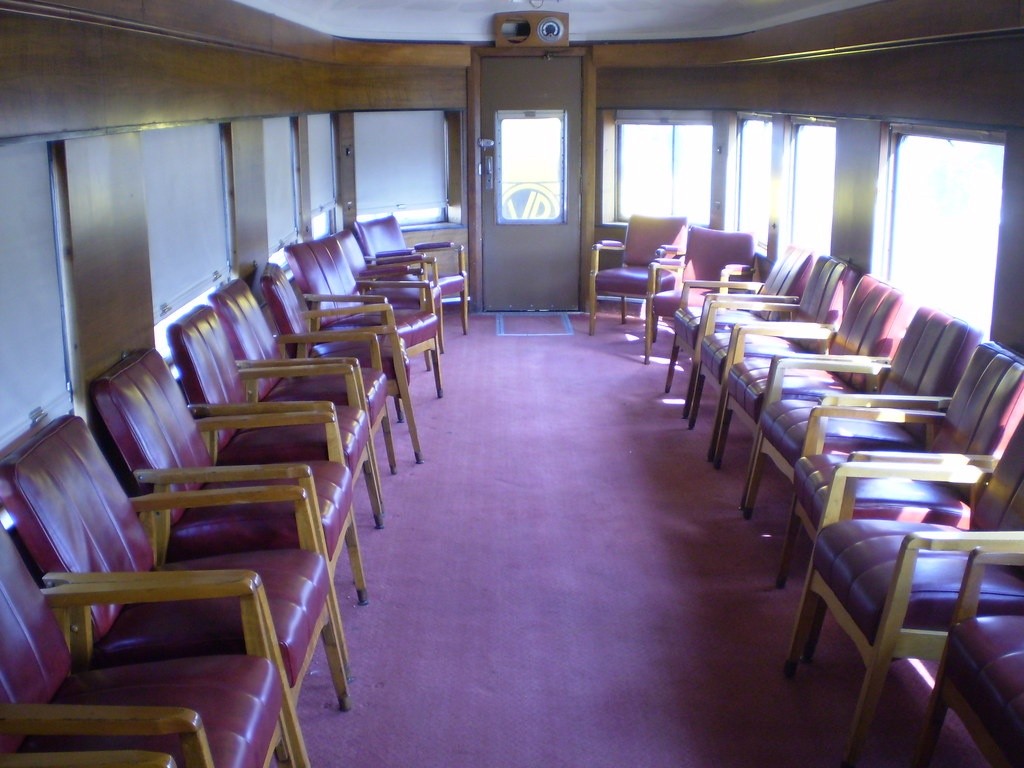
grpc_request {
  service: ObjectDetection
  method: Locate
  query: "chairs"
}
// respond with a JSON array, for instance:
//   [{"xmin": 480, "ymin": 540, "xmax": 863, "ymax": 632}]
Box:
[
  {"xmin": 0, "ymin": 215, "xmax": 468, "ymax": 768},
  {"xmin": 586, "ymin": 214, "xmax": 1024, "ymax": 768}
]
